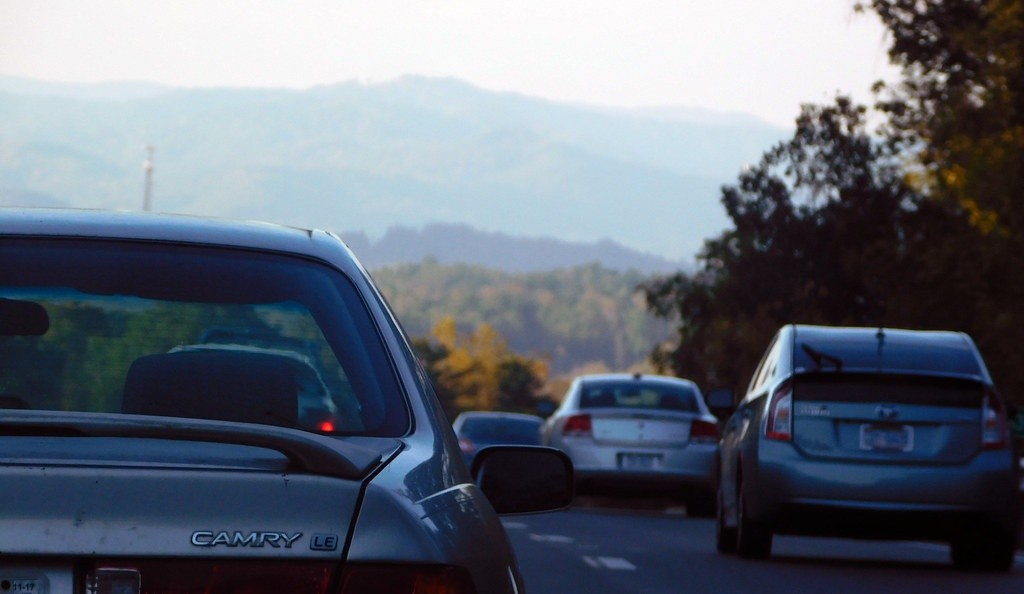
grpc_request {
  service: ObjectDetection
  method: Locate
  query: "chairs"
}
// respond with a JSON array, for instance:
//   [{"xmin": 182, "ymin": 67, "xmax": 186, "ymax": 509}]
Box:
[{"xmin": 122, "ymin": 346, "xmax": 337, "ymax": 434}]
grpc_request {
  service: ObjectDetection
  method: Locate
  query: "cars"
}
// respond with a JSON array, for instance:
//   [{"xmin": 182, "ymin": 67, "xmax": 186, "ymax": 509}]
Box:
[
  {"xmin": 713, "ymin": 323, "xmax": 1023, "ymax": 574},
  {"xmin": 541, "ymin": 372, "xmax": 722, "ymax": 520},
  {"xmin": 0, "ymin": 207, "xmax": 578, "ymax": 594},
  {"xmin": 169, "ymin": 344, "xmax": 344, "ymax": 434},
  {"xmin": 452, "ymin": 411, "xmax": 544, "ymax": 469}
]
